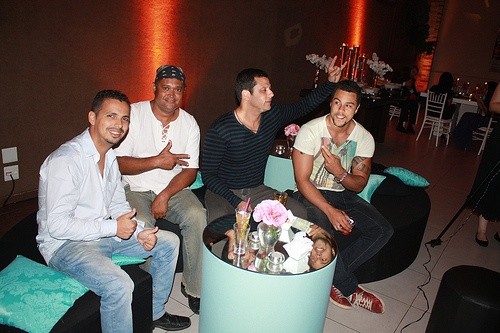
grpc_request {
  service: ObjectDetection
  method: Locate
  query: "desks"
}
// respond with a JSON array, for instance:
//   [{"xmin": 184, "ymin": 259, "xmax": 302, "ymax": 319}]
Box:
[
  {"xmin": 370, "ymin": 81, "xmax": 401, "ymax": 98},
  {"xmin": 419, "ymin": 91, "xmax": 483, "ymax": 126},
  {"xmin": 296, "ymin": 88, "xmax": 391, "ymax": 146}
]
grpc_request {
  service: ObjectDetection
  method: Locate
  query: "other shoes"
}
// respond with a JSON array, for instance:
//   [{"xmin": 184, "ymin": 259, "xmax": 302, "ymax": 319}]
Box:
[
  {"xmin": 396, "ymin": 126, "xmax": 406, "ymax": 132},
  {"xmin": 494, "ymin": 232, "xmax": 500, "ymax": 241},
  {"xmin": 475, "ymin": 231, "xmax": 488, "ymax": 247},
  {"xmin": 406, "ymin": 126, "xmax": 416, "ymax": 134}
]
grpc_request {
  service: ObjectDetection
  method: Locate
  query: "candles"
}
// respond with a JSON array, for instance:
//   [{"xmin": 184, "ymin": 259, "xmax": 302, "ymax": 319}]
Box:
[
  {"xmin": 340, "ymin": 42, "xmax": 348, "ymax": 76},
  {"xmin": 348, "ymin": 44, "xmax": 360, "ymax": 79},
  {"xmin": 357, "ymin": 55, "xmax": 368, "ymax": 80}
]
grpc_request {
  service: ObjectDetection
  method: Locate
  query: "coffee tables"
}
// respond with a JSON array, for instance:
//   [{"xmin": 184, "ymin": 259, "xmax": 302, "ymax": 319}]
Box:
[{"xmin": 198, "ymin": 212, "xmax": 339, "ymax": 333}]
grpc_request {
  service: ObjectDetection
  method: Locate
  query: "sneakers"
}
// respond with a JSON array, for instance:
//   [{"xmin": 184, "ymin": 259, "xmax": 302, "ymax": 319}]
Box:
[
  {"xmin": 347, "ymin": 285, "xmax": 385, "ymax": 314},
  {"xmin": 330, "ymin": 284, "xmax": 353, "ymax": 308}
]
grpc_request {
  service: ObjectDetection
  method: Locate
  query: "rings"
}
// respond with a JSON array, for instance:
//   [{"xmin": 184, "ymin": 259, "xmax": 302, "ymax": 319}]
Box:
[{"xmin": 337, "ymin": 225, "xmax": 341, "ymax": 228}]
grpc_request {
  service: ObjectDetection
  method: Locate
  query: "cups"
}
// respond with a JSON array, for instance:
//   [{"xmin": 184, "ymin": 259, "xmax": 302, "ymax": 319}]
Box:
[
  {"xmin": 233, "ymin": 222, "xmax": 251, "ymax": 255},
  {"xmin": 235, "ymin": 207, "xmax": 251, "ymax": 242},
  {"xmin": 266, "ymin": 252, "xmax": 285, "ymax": 272},
  {"xmin": 341, "ymin": 217, "xmax": 356, "ymax": 235},
  {"xmin": 248, "ymin": 231, "xmax": 261, "ymax": 250}
]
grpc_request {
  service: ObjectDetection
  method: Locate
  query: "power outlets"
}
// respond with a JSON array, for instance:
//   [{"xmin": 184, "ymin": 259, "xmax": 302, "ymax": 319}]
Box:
[{"xmin": 3, "ymin": 165, "xmax": 20, "ymax": 181}]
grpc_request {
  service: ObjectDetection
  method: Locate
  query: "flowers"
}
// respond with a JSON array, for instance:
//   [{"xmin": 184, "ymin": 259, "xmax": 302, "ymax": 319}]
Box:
[
  {"xmin": 284, "ymin": 124, "xmax": 301, "ymax": 160},
  {"xmin": 366, "ymin": 52, "xmax": 394, "ymax": 78},
  {"xmin": 252, "ymin": 199, "xmax": 289, "ymax": 266},
  {"xmin": 305, "ymin": 53, "xmax": 333, "ymax": 73}
]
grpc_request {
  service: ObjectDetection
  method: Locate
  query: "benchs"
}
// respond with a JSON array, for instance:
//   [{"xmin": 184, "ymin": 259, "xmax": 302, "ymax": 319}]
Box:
[{"xmin": 0, "ymin": 160, "xmax": 431, "ymax": 333}]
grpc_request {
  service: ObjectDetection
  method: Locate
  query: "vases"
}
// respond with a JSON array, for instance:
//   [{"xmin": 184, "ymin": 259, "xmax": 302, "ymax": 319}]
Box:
[{"xmin": 254, "ymin": 221, "xmax": 283, "ymax": 272}]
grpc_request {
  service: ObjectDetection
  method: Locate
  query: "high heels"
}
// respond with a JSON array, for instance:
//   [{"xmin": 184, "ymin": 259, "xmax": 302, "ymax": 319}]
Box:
[{"xmin": 441, "ymin": 134, "xmax": 447, "ymax": 142}]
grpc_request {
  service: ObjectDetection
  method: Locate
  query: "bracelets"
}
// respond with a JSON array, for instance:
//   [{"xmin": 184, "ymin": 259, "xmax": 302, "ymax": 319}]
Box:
[{"xmin": 334, "ymin": 169, "xmax": 348, "ymax": 184}]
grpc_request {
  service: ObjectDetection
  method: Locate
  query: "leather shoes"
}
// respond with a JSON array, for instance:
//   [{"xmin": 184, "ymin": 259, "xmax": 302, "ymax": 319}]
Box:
[
  {"xmin": 152, "ymin": 312, "xmax": 192, "ymax": 331},
  {"xmin": 180, "ymin": 281, "xmax": 201, "ymax": 314}
]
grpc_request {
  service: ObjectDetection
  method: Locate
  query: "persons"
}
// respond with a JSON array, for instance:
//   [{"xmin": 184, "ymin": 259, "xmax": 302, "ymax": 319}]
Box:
[
  {"xmin": 391, "ymin": 65, "xmax": 419, "ymax": 134},
  {"xmin": 292, "ymin": 79, "xmax": 395, "ymax": 315},
  {"xmin": 200, "ymin": 55, "xmax": 347, "ymax": 221},
  {"xmin": 207, "ymin": 215, "xmax": 261, "ymax": 270},
  {"xmin": 306, "ymin": 224, "xmax": 336, "ymax": 270},
  {"xmin": 452, "ymin": 81, "xmax": 500, "ymax": 152},
  {"xmin": 36, "ymin": 89, "xmax": 192, "ymax": 333},
  {"xmin": 427, "ymin": 72, "xmax": 457, "ymax": 120},
  {"xmin": 465, "ymin": 83, "xmax": 500, "ymax": 248},
  {"xmin": 111, "ymin": 65, "xmax": 208, "ymax": 315}
]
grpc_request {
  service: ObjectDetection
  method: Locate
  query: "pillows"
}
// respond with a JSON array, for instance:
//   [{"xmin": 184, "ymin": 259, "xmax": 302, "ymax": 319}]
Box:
[
  {"xmin": 0, "ymin": 255, "xmax": 91, "ymax": 333},
  {"xmin": 356, "ymin": 175, "xmax": 387, "ymax": 204},
  {"xmin": 189, "ymin": 170, "xmax": 204, "ymax": 189},
  {"xmin": 382, "ymin": 167, "xmax": 430, "ymax": 187},
  {"xmin": 111, "ymin": 254, "xmax": 147, "ymax": 266}
]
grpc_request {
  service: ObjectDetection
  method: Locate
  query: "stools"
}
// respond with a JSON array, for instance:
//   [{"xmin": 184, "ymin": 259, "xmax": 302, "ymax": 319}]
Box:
[{"xmin": 425, "ymin": 265, "xmax": 500, "ymax": 333}]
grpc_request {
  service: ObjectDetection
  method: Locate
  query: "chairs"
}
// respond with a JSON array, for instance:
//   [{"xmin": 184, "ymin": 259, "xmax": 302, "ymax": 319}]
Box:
[
  {"xmin": 472, "ymin": 117, "xmax": 497, "ymax": 156},
  {"xmin": 414, "ymin": 89, "xmax": 453, "ymax": 147},
  {"xmin": 389, "ymin": 87, "xmax": 405, "ymax": 120}
]
